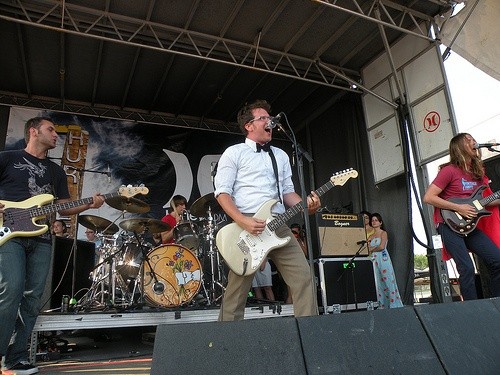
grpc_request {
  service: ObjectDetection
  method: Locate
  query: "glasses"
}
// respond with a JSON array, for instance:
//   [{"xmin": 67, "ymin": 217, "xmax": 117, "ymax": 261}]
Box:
[{"xmin": 249, "ymin": 116, "xmax": 272, "ymax": 123}]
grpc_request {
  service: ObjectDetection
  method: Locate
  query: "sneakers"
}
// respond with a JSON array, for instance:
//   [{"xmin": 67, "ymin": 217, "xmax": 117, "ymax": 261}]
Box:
[{"xmin": 4, "ymin": 360, "xmax": 39, "ymax": 375}]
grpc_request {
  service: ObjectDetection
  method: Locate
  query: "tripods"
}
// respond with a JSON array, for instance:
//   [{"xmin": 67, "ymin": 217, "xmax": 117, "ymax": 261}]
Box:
[{"xmin": 44, "ymin": 170, "xmax": 85, "ymax": 315}]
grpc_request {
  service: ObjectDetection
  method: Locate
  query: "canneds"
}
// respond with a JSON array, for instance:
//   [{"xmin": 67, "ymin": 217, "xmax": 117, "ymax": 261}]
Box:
[{"xmin": 61, "ymin": 295, "xmax": 70, "ymax": 313}]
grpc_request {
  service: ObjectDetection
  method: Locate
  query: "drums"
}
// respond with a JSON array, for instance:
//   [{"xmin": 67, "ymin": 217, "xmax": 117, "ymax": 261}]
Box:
[
  {"xmin": 136, "ymin": 243, "xmax": 204, "ymax": 309},
  {"xmin": 172, "ymin": 221, "xmax": 200, "ymax": 250},
  {"xmin": 115, "ymin": 242, "xmax": 151, "ymax": 277}
]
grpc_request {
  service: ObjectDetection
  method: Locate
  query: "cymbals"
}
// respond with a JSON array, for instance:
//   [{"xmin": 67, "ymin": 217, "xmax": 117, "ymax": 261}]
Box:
[
  {"xmin": 105, "ymin": 196, "xmax": 151, "ymax": 214},
  {"xmin": 190, "ymin": 192, "xmax": 220, "ymax": 217},
  {"xmin": 77, "ymin": 215, "xmax": 119, "ymax": 234},
  {"xmin": 119, "ymin": 218, "xmax": 170, "ymax": 233}
]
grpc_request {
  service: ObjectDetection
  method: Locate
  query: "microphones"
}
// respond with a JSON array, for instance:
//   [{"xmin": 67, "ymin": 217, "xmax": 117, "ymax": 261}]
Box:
[
  {"xmin": 268, "ymin": 113, "xmax": 282, "ymax": 129},
  {"xmin": 474, "ymin": 142, "xmax": 500, "ymax": 148},
  {"xmin": 107, "ymin": 166, "xmax": 112, "ymax": 183},
  {"xmin": 356, "ymin": 240, "xmax": 371, "ymax": 245}
]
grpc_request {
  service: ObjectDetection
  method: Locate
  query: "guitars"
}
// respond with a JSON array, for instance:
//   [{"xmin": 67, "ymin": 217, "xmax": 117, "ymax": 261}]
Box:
[
  {"xmin": 440, "ymin": 184, "xmax": 500, "ymax": 235},
  {"xmin": 0, "ymin": 184, "xmax": 149, "ymax": 246},
  {"xmin": 216, "ymin": 168, "xmax": 358, "ymax": 277}
]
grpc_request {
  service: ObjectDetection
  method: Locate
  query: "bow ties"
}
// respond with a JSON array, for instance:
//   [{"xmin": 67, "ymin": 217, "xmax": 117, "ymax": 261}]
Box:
[{"xmin": 255, "ymin": 142, "xmax": 270, "ymax": 152}]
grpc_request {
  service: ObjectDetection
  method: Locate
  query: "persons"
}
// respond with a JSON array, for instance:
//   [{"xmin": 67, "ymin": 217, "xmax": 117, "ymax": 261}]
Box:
[
  {"xmin": 52, "ymin": 219, "xmax": 68, "ymax": 238},
  {"xmin": 250, "ymin": 256, "xmax": 275, "ymax": 302},
  {"xmin": 0, "ymin": 115, "xmax": 104, "ymax": 375},
  {"xmin": 360, "ymin": 210, "xmax": 375, "ymax": 246},
  {"xmin": 367, "ymin": 212, "xmax": 404, "ymax": 310},
  {"xmin": 215, "ymin": 98, "xmax": 321, "ymax": 325},
  {"xmin": 84, "ymin": 227, "xmax": 101, "ymax": 262},
  {"xmin": 286, "ymin": 222, "xmax": 306, "ymax": 305},
  {"xmin": 160, "ymin": 195, "xmax": 187, "ymax": 245},
  {"xmin": 423, "ymin": 132, "xmax": 500, "ymax": 301}
]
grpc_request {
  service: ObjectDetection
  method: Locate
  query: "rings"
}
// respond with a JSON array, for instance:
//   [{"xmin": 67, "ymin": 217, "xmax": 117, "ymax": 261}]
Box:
[{"xmin": 256, "ymin": 231, "xmax": 260, "ymax": 234}]
